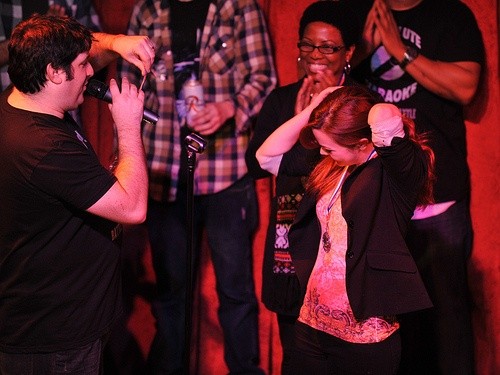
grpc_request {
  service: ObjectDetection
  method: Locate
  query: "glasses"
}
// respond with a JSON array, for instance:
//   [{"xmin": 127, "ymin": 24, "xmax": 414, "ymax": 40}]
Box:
[{"xmin": 297, "ymin": 41, "xmax": 346, "ymax": 56}]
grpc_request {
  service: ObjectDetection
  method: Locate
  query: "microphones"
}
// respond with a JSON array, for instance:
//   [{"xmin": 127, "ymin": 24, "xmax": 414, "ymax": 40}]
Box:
[{"xmin": 86, "ymin": 79, "xmax": 160, "ymax": 125}]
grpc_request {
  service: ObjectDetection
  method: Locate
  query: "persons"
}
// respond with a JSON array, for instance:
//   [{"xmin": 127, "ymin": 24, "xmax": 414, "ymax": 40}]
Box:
[
  {"xmin": 351, "ymin": 0, "xmax": 487, "ymax": 375},
  {"xmin": 110, "ymin": 0, "xmax": 277, "ymax": 375},
  {"xmin": 256, "ymin": 85, "xmax": 436, "ymax": 375},
  {"xmin": 1, "ymin": 12, "xmax": 156, "ymax": 375},
  {"xmin": 0, "ymin": 0, "xmax": 104, "ymax": 134},
  {"xmin": 245, "ymin": 0, "xmax": 384, "ymax": 375}
]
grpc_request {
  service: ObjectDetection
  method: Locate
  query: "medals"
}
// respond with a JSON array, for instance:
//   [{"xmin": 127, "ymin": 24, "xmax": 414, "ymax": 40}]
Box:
[{"xmin": 323, "ymin": 232, "xmax": 331, "ymax": 253}]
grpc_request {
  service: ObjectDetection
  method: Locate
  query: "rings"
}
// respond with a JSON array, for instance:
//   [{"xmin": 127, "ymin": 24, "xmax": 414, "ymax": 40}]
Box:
[{"xmin": 150, "ymin": 46, "xmax": 155, "ymax": 49}]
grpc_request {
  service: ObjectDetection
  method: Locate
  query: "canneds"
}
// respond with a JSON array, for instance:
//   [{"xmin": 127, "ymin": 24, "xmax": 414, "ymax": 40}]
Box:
[{"xmin": 182, "ymin": 80, "xmax": 205, "ymax": 128}]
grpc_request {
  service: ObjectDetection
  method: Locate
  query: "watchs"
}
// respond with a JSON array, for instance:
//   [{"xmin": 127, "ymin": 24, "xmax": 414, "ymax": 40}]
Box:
[{"xmin": 400, "ymin": 45, "xmax": 419, "ymax": 69}]
